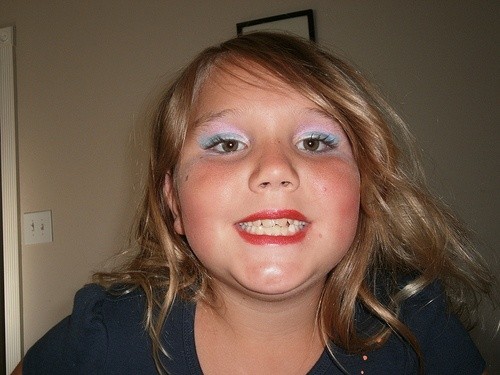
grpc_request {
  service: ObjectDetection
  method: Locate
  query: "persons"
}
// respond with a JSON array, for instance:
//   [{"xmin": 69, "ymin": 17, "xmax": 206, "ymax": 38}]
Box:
[{"xmin": 11, "ymin": 32, "xmax": 494, "ymax": 375}]
